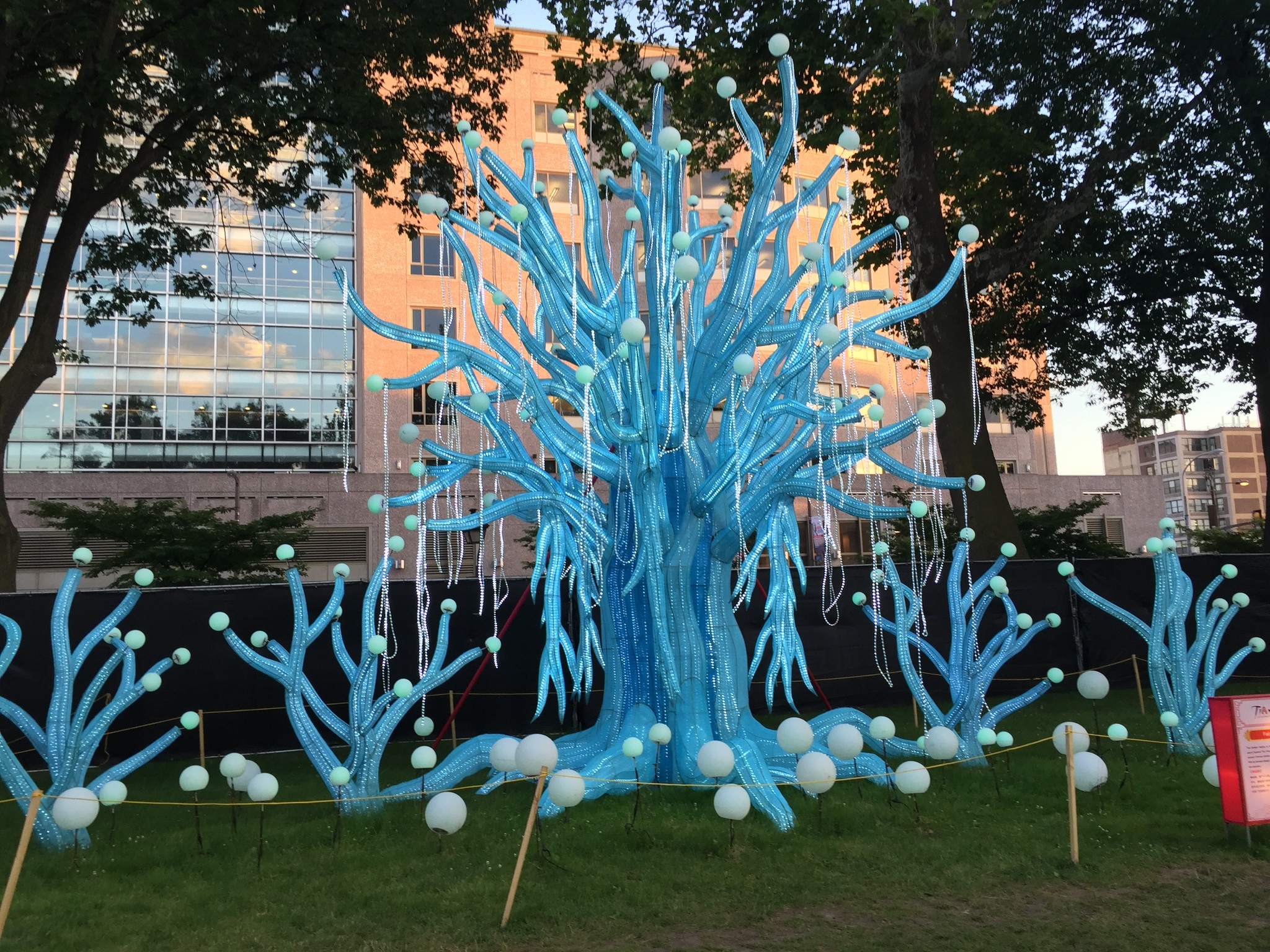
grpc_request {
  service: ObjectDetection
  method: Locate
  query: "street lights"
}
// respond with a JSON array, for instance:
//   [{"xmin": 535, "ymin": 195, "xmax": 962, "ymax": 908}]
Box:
[
  {"xmin": 1181, "ymin": 449, "xmax": 1225, "ymax": 554},
  {"xmin": 1214, "ymin": 478, "xmax": 1250, "ymax": 527}
]
